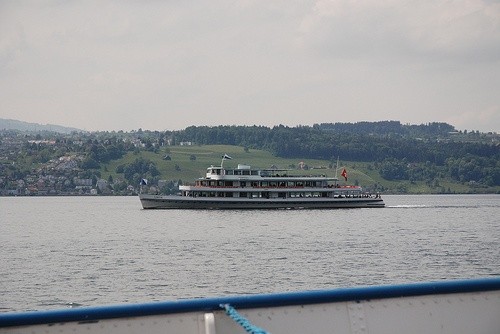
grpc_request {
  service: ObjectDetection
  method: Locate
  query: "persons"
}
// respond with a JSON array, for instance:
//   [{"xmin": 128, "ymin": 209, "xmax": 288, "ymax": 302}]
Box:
[{"xmin": 327, "ymin": 184, "xmax": 340, "ymax": 188}]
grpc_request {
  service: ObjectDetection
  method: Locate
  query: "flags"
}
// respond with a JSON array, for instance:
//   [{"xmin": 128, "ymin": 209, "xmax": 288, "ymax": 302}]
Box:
[
  {"xmin": 341, "ymin": 169, "xmax": 347, "ymax": 182},
  {"xmin": 221, "ymin": 154, "xmax": 232, "ymax": 160}
]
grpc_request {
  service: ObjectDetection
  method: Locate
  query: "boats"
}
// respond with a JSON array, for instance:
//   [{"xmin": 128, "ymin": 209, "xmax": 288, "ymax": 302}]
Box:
[{"xmin": 138, "ymin": 153, "xmax": 386, "ymax": 210}]
